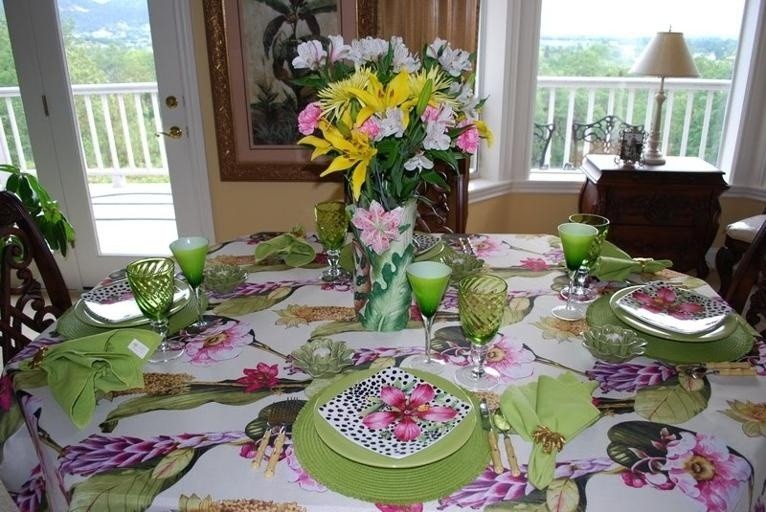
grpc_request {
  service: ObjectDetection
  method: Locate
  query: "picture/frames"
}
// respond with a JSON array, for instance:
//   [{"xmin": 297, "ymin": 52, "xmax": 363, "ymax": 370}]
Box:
[{"xmin": 200, "ymin": 1, "xmax": 375, "ymax": 183}]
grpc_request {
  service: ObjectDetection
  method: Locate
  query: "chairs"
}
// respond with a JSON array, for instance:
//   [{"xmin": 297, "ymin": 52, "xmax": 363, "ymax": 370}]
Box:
[
  {"xmin": 0, "ymin": 189, "xmax": 74, "ymax": 379},
  {"xmin": 530, "ymin": 121, "xmax": 554, "ymax": 170},
  {"xmin": 560, "ymin": 115, "xmax": 644, "ymax": 174}
]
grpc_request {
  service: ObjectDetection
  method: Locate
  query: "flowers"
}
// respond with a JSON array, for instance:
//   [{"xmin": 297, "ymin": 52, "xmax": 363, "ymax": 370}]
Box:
[{"xmin": 276, "ymin": 32, "xmax": 497, "ymax": 253}]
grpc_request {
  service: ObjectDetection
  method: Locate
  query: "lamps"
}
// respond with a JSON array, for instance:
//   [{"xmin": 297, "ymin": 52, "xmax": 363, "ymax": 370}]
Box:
[{"xmin": 628, "ymin": 21, "xmax": 703, "ymax": 165}]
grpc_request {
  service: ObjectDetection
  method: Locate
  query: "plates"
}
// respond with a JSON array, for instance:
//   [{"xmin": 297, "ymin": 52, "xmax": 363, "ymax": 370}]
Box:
[
  {"xmin": 290, "ymin": 339, "xmax": 355, "ymax": 379},
  {"xmin": 411, "ymin": 241, "xmax": 446, "ymax": 262},
  {"xmin": 440, "ymin": 251, "xmax": 486, "ymax": 281},
  {"xmin": 74, "ymin": 276, "xmax": 193, "ymax": 329},
  {"xmin": 608, "ymin": 285, "xmax": 739, "ymax": 342},
  {"xmin": 581, "ymin": 323, "xmax": 648, "ymax": 363},
  {"xmin": 311, "ymin": 368, "xmax": 477, "ymax": 469},
  {"xmin": 203, "ymin": 263, "xmax": 248, "ymax": 295}
]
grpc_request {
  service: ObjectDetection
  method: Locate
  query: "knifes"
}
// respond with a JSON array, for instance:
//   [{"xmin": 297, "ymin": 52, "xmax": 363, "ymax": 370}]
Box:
[{"xmin": 480, "ymin": 397, "xmax": 504, "ymax": 474}]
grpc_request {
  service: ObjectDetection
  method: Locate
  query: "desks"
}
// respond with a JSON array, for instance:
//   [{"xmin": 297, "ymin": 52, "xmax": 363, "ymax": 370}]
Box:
[
  {"xmin": 579, "ymin": 154, "xmax": 729, "ymax": 276},
  {"xmin": 0, "ymin": 229, "xmax": 766, "ymax": 511}
]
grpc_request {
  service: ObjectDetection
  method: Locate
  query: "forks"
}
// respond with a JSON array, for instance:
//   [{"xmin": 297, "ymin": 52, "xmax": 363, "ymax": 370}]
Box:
[
  {"xmin": 658, "ymin": 359, "xmax": 758, "ymax": 379},
  {"xmin": 264, "ymin": 396, "xmax": 300, "ymax": 478},
  {"xmin": 250, "ymin": 402, "xmax": 284, "ymax": 471}
]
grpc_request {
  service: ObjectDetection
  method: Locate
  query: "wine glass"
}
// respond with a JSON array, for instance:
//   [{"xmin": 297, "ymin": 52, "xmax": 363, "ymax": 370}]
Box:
[
  {"xmin": 314, "ymin": 200, "xmax": 351, "ymax": 283},
  {"xmin": 551, "ymin": 223, "xmax": 599, "ymax": 321},
  {"xmin": 127, "ymin": 257, "xmax": 184, "ymax": 362},
  {"xmin": 169, "ymin": 236, "xmax": 214, "ymax": 330},
  {"xmin": 405, "ymin": 261, "xmax": 453, "ymax": 374},
  {"xmin": 455, "ymin": 273, "xmax": 507, "ymax": 392},
  {"xmin": 560, "ymin": 213, "xmax": 610, "ymax": 303}
]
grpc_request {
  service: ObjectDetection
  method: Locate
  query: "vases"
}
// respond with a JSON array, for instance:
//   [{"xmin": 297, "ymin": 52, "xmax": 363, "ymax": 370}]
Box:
[{"xmin": 348, "ymin": 200, "xmax": 415, "ymax": 330}]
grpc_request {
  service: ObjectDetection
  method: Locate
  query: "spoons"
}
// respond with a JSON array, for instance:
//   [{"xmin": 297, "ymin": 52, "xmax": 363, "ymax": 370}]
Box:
[{"xmin": 493, "ymin": 407, "xmax": 521, "ymax": 477}]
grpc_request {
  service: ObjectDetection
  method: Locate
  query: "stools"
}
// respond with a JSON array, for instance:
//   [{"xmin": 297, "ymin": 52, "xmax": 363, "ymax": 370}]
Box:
[{"xmin": 715, "ymin": 211, "xmax": 766, "ymax": 296}]
